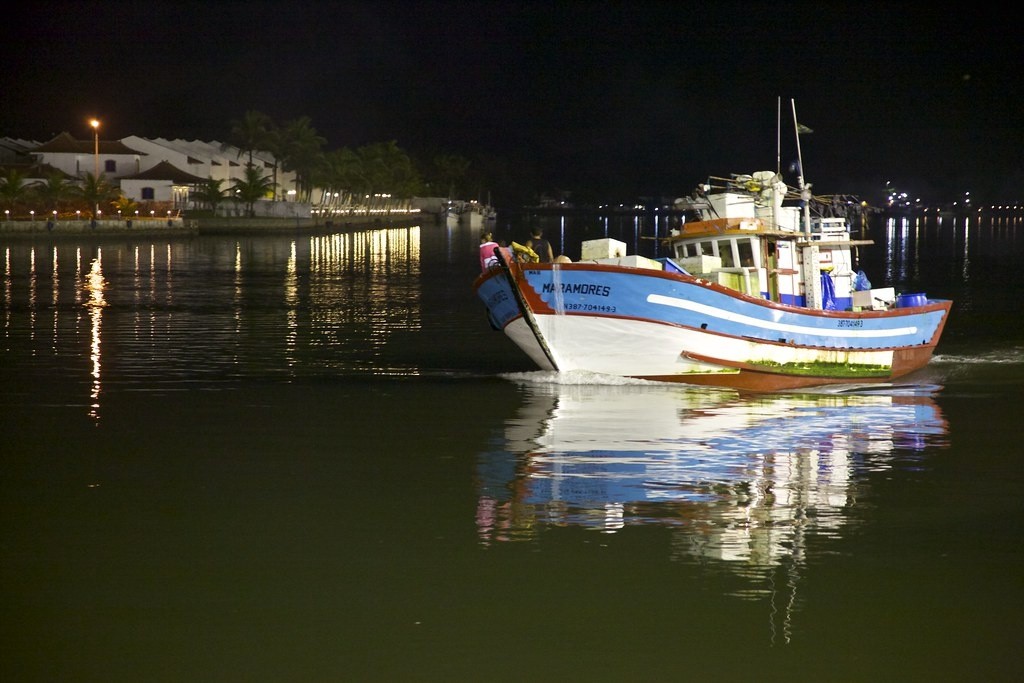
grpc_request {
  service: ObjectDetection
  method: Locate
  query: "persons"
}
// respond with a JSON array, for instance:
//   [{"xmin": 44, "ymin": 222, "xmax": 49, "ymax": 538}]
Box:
[
  {"xmin": 479, "ymin": 232, "xmax": 500, "ymax": 272},
  {"xmin": 527, "ymin": 225, "xmax": 553, "ymax": 264}
]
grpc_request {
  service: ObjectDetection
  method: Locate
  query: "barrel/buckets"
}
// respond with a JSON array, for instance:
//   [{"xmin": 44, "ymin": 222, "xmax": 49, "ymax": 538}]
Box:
[{"xmin": 895, "ymin": 292, "xmax": 927, "ymax": 308}]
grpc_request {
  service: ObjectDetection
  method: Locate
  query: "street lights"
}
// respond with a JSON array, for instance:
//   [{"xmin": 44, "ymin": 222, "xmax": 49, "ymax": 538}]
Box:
[{"xmin": 87, "ymin": 116, "xmax": 101, "ymax": 219}]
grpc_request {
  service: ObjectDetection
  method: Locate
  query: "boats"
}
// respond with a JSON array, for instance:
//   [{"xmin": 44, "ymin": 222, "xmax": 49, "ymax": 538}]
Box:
[
  {"xmin": 476, "ymin": 366, "xmax": 953, "ymax": 645},
  {"xmin": 469, "ymin": 95, "xmax": 955, "ymax": 394}
]
